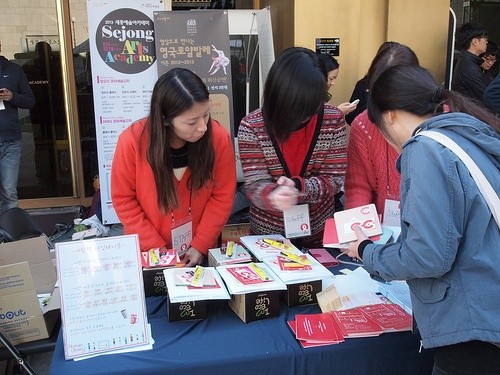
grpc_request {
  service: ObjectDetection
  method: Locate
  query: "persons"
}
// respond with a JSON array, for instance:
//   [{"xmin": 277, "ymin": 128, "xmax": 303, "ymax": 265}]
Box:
[
  {"xmin": 237, "ymin": 47, "xmax": 347, "ymax": 249},
  {"xmin": 22, "ymin": 42, "xmax": 68, "ymax": 181},
  {"xmin": 484, "ymin": 73, "xmax": 500, "ymax": 114},
  {"xmin": 479, "ymin": 40, "xmax": 500, "ymax": 83},
  {"xmin": 319, "ymin": 53, "xmax": 356, "ymax": 115},
  {"xmin": 208, "ymin": 45, "xmax": 229, "ymax": 75},
  {"xmin": 452, "ymin": 21, "xmax": 490, "ymax": 101},
  {"xmin": 345, "ymin": 41, "xmax": 399, "ymax": 127},
  {"xmin": 348, "ymin": 64, "xmax": 500, "ymax": 375},
  {"xmin": 344, "ymin": 44, "xmax": 419, "ymax": 221},
  {"xmin": 0, "ymin": 55, "xmax": 36, "ymax": 211},
  {"xmin": 111, "ymin": 68, "xmax": 236, "ymax": 267}
]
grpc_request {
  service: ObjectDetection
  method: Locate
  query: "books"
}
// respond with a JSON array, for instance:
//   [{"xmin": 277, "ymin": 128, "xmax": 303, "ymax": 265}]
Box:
[
  {"xmin": 323, "ymin": 214, "xmax": 382, "ymax": 249},
  {"xmin": 378, "ymin": 280, "xmax": 413, "ymax": 316},
  {"xmin": 287, "ymin": 303, "xmax": 413, "ymax": 349}
]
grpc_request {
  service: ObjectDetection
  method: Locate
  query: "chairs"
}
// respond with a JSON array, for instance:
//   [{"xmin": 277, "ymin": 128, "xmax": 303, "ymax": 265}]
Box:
[{"xmin": 0, "ymin": 208, "xmax": 55, "ymax": 249}]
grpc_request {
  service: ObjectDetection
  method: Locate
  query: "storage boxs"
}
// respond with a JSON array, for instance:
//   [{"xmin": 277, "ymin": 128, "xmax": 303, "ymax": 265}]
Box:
[
  {"xmin": 227, "ymin": 290, "xmax": 283, "ymax": 324},
  {"xmin": 0, "ymin": 235, "xmax": 63, "ymax": 347},
  {"xmin": 166, "ymin": 292, "xmax": 209, "ymax": 323},
  {"xmin": 142, "ymin": 265, "xmax": 168, "ymax": 297},
  {"xmin": 284, "ymin": 280, "xmax": 323, "ymax": 307},
  {"xmin": 208, "ymin": 252, "xmax": 221, "ymax": 268}
]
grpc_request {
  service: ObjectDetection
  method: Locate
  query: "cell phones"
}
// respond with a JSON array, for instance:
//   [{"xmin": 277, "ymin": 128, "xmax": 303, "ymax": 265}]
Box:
[
  {"xmin": 348, "ymin": 99, "xmax": 360, "ymax": 106},
  {"xmin": 0, "ymin": 88, "xmax": 7, "ymax": 94}
]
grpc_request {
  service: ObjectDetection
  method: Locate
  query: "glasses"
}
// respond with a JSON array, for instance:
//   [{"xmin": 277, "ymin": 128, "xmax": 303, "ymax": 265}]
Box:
[
  {"xmin": 482, "ymin": 55, "xmax": 497, "ymax": 64},
  {"xmin": 480, "ymin": 35, "xmax": 490, "ymax": 41}
]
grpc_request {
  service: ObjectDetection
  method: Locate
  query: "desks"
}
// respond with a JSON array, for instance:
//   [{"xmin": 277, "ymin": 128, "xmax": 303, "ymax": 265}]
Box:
[{"xmin": 50, "ymin": 247, "xmax": 434, "ymax": 375}]
